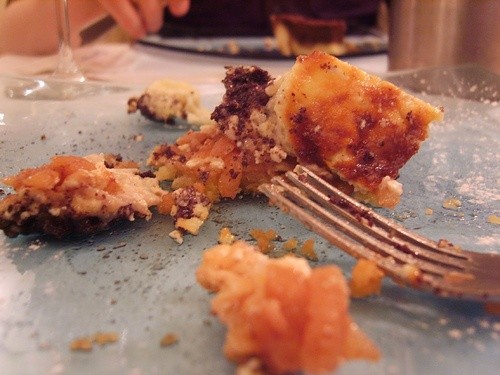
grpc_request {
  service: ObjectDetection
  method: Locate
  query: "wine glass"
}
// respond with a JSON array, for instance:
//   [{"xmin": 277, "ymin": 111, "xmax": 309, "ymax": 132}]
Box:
[{"xmin": 4, "ymin": 0, "xmax": 129, "ymax": 100}]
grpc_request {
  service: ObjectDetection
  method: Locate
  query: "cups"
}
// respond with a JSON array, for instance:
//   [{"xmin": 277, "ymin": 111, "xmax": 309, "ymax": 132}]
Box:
[{"xmin": 382, "ymin": 0, "xmax": 500, "ymax": 104}]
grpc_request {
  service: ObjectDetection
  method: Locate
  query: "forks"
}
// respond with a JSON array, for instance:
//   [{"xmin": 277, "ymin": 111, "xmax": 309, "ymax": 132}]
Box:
[{"xmin": 255, "ymin": 165, "xmax": 500, "ymax": 307}]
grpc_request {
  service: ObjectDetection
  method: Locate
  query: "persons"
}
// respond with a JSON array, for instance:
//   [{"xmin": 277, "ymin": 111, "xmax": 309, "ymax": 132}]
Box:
[{"xmin": 0, "ymin": 0, "xmax": 390, "ymax": 59}]
